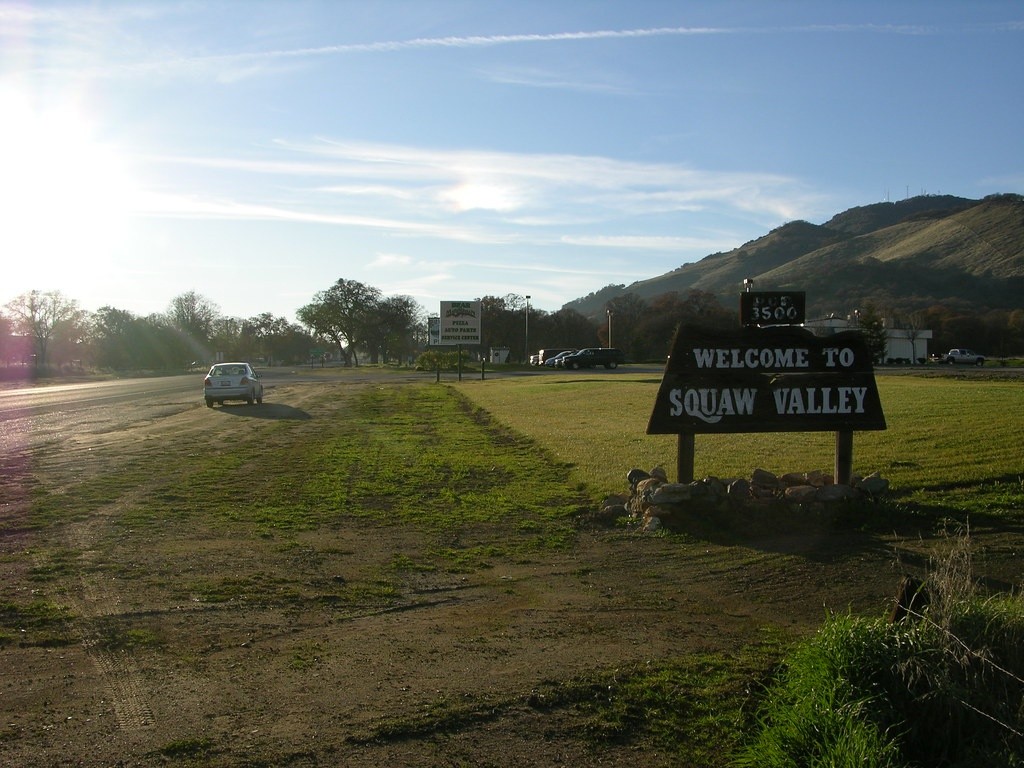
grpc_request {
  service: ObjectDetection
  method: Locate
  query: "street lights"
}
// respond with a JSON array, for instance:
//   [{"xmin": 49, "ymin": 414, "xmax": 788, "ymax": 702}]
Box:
[
  {"xmin": 606, "ymin": 309, "xmax": 614, "ymax": 349},
  {"xmin": 525, "ymin": 295, "xmax": 531, "ymax": 363}
]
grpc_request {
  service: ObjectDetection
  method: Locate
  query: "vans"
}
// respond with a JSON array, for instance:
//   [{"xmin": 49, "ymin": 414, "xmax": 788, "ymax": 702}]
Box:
[{"xmin": 539, "ymin": 349, "xmax": 565, "ymax": 366}]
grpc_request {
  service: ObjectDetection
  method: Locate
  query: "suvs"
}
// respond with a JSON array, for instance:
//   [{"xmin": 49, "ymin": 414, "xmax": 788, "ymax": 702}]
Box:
[{"xmin": 562, "ymin": 348, "xmax": 626, "ymax": 371}]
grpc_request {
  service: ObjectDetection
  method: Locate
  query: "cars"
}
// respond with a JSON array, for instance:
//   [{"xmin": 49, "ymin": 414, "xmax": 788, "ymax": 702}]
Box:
[
  {"xmin": 554, "ymin": 350, "xmax": 581, "ymax": 369},
  {"xmin": 204, "ymin": 362, "xmax": 263, "ymax": 407},
  {"xmin": 545, "ymin": 350, "xmax": 573, "ymax": 367}
]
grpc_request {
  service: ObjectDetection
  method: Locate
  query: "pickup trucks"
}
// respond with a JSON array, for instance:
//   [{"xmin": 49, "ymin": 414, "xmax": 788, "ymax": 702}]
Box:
[{"xmin": 942, "ymin": 348, "xmax": 985, "ymax": 366}]
